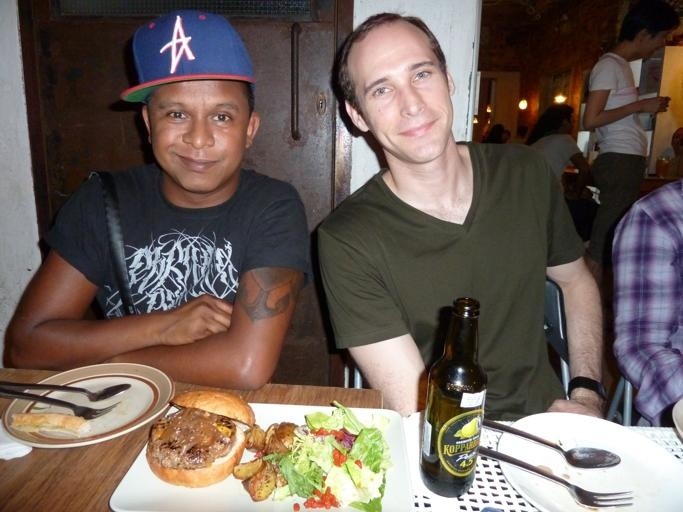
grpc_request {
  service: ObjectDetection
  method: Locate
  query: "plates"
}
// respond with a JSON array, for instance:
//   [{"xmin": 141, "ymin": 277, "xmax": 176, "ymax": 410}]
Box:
[
  {"xmin": 497, "ymin": 412, "xmax": 683, "ymax": 510},
  {"xmin": 670, "ymin": 395, "xmax": 683, "ymax": 441},
  {"xmin": 107, "ymin": 401, "xmax": 408, "ymax": 512},
  {"xmin": 3, "ymin": 361, "xmax": 176, "ymax": 450}
]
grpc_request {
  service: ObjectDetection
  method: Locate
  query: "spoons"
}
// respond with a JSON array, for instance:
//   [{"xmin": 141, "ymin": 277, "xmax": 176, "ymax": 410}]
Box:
[
  {"xmin": 480, "ymin": 408, "xmax": 622, "ymax": 471},
  {"xmin": 0, "ymin": 376, "xmax": 129, "ymax": 401}
]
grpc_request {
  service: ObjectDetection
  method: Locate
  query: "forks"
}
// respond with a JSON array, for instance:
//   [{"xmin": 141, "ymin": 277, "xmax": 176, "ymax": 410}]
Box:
[
  {"xmin": 476, "ymin": 446, "xmax": 640, "ymax": 512},
  {"xmin": 0, "ymin": 385, "xmax": 119, "ymax": 422}
]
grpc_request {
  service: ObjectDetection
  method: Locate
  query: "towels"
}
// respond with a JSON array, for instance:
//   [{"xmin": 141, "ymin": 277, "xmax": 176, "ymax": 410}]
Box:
[{"xmin": 0, "ymin": 418, "xmax": 34, "ymax": 460}]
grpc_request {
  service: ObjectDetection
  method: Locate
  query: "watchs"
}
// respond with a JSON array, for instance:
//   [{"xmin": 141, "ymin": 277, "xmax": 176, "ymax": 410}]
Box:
[{"xmin": 565, "ymin": 375, "xmax": 607, "ymax": 402}]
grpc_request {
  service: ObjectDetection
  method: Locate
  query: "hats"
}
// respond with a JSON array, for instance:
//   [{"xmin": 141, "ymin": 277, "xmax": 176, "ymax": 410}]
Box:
[{"xmin": 118, "ymin": 8, "xmax": 259, "ymax": 104}]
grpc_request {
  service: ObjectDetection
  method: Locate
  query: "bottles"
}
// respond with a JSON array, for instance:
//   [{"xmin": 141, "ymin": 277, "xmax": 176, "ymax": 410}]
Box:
[
  {"xmin": 657, "ymin": 153, "xmax": 670, "ymax": 179},
  {"xmin": 418, "ymin": 297, "xmax": 491, "ymax": 500}
]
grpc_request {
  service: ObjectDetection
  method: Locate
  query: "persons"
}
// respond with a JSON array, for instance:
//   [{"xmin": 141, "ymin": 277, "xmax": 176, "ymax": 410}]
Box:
[
  {"xmin": 580, "ymin": 1, "xmax": 680, "ymax": 264},
  {"xmin": 480, "ymin": 123, "xmax": 528, "ymax": 147},
  {"xmin": 610, "ymin": 177, "xmax": 683, "ymax": 437},
  {"xmin": 315, "ymin": 12, "xmax": 607, "ymax": 421},
  {"xmin": 523, "ymin": 102, "xmax": 596, "ymax": 241},
  {"xmin": 2, "ymin": 8, "xmax": 313, "ymax": 386}
]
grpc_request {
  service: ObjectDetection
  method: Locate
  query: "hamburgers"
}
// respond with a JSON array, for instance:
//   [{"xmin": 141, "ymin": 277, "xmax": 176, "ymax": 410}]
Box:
[{"xmin": 145, "ymin": 390, "xmax": 256, "ymax": 487}]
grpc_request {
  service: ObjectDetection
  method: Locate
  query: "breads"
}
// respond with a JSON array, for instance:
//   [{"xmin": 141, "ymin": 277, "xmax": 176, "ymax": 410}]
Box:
[{"xmin": 11, "ymin": 411, "xmax": 85, "ymax": 436}]
